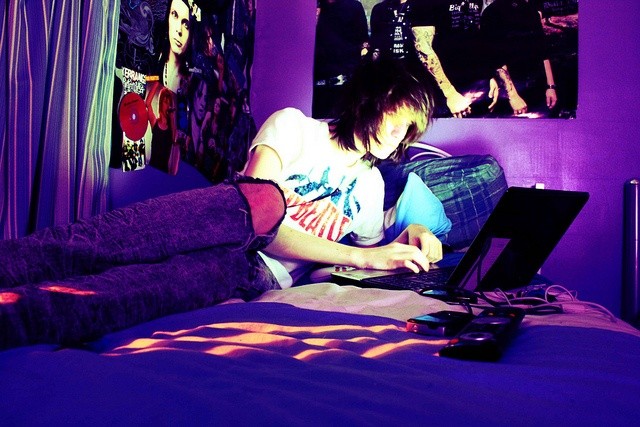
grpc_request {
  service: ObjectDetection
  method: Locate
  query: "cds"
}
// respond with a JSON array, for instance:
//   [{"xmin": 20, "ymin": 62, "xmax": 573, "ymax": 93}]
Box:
[{"xmin": 119, "ymin": 94, "xmax": 148, "ymax": 141}]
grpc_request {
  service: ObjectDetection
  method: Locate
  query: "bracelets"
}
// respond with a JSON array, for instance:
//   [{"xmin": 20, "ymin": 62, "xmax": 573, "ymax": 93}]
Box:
[{"xmin": 546, "ymin": 84, "xmax": 556, "ymax": 89}]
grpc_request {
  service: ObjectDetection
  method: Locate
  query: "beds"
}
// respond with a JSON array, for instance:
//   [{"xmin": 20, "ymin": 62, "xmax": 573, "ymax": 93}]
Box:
[{"xmin": 1, "ymin": 143, "xmax": 639, "ymax": 427}]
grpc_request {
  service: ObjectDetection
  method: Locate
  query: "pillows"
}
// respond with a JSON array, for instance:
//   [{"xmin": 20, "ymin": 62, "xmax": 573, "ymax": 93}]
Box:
[{"xmin": 338, "ymin": 155, "xmax": 508, "ymax": 251}]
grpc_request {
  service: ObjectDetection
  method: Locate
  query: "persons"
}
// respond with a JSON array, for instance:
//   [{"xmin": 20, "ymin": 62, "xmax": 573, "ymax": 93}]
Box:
[
  {"xmin": 145, "ymin": 82, "xmax": 180, "ymax": 175},
  {"xmin": 1, "ymin": 75, "xmax": 443, "ymax": 352},
  {"xmin": 152, "ymin": 0, "xmax": 201, "ymax": 95},
  {"xmin": 198, "ymin": 97, "xmax": 222, "ymax": 156},
  {"xmin": 483, "ymin": 0, "xmax": 557, "ymax": 114},
  {"xmin": 184, "ymin": 72, "xmax": 207, "ymax": 153},
  {"xmin": 370, "ymin": 0, "xmax": 406, "ymax": 88},
  {"xmin": 406, "ymin": 1, "xmax": 499, "ymax": 117}
]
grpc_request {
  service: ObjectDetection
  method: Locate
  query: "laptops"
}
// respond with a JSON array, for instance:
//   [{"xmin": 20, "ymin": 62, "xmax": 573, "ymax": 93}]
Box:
[{"xmin": 330, "ymin": 188, "xmax": 590, "ymax": 292}]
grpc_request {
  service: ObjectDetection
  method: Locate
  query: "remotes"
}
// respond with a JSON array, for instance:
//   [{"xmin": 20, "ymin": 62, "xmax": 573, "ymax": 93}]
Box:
[{"xmin": 439, "ymin": 307, "xmax": 525, "ymax": 360}]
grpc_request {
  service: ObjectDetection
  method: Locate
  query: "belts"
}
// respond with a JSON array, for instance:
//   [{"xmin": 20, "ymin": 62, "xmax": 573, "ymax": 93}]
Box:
[{"xmin": 257, "ymin": 251, "xmax": 295, "ymax": 291}]
